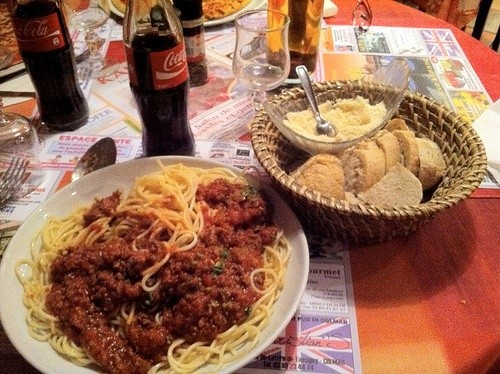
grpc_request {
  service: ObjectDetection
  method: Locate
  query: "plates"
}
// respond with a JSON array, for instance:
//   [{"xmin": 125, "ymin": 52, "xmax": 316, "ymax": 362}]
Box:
[
  {"xmin": 0, "ymin": 64, "xmax": 26, "ymax": 79},
  {"xmin": 107, "ymin": 0, "xmax": 265, "ymax": 27}
]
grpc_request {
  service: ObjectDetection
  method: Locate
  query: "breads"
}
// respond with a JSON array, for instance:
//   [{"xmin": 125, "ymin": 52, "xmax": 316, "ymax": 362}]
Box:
[{"xmin": 291, "ymin": 119, "xmax": 446, "ymax": 207}]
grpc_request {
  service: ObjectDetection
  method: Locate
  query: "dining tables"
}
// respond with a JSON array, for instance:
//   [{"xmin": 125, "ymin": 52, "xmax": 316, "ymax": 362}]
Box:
[{"xmin": 0, "ymin": 0, "xmax": 500, "ymax": 374}]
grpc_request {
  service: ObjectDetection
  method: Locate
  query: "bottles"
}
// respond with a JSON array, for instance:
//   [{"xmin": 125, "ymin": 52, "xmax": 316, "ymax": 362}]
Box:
[
  {"xmin": 266, "ymin": 0, "xmax": 325, "ymax": 96},
  {"xmin": 7, "ymin": 0, "xmax": 90, "ymax": 132},
  {"xmin": 122, "ymin": 0, "xmax": 196, "ymax": 156},
  {"xmin": 172, "ymin": 0, "xmax": 209, "ymax": 86}
]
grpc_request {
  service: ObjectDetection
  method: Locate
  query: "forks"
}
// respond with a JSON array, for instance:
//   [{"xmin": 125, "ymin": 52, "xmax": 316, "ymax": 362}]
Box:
[{"xmin": 0, "ymin": 156, "xmax": 28, "ymax": 211}]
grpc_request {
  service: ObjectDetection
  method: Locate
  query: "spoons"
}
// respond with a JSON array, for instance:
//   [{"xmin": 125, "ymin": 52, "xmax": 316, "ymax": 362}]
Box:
[
  {"xmin": 296, "ymin": 65, "xmax": 337, "ymax": 138},
  {"xmin": 70, "ymin": 136, "xmax": 117, "ymax": 183}
]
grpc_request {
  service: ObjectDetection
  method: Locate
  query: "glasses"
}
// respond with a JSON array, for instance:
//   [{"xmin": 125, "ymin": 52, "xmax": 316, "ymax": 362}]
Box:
[{"xmin": 351, "ymin": 0, "xmax": 372, "ymax": 39}]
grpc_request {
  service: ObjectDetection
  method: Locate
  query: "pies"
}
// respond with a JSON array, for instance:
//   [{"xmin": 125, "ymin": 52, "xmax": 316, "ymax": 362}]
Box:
[
  {"xmin": 0, "ymin": 0, "xmax": 91, "ymax": 66},
  {"xmin": 110, "ymin": 0, "xmax": 252, "ymax": 22}
]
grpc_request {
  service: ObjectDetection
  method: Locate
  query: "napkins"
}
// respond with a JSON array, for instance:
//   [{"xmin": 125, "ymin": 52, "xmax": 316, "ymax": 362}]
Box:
[{"xmin": 471, "ymin": 99, "xmax": 500, "ymax": 185}]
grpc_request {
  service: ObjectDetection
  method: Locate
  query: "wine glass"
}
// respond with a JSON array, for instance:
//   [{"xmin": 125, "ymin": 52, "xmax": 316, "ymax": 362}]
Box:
[
  {"xmin": 231, "ymin": 9, "xmax": 291, "ymax": 145},
  {"xmin": 59, "ymin": 0, "xmax": 121, "ymax": 77},
  {"xmin": 0, "ymin": 96, "xmax": 43, "ymax": 200}
]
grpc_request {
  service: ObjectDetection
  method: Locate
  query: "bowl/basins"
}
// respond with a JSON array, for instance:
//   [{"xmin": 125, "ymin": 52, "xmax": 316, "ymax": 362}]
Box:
[
  {"xmin": 263, "ymin": 59, "xmax": 415, "ymax": 154},
  {"xmin": 0, "ymin": 154, "xmax": 310, "ymax": 374}
]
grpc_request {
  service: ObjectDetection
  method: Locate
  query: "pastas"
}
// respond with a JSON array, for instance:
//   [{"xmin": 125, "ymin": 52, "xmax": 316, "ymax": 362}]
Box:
[{"xmin": 15, "ymin": 160, "xmax": 294, "ymax": 374}]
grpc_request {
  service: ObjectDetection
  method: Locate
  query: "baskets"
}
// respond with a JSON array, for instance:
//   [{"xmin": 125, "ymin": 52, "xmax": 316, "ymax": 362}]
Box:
[{"xmin": 250, "ymin": 78, "xmax": 489, "ymax": 248}]
